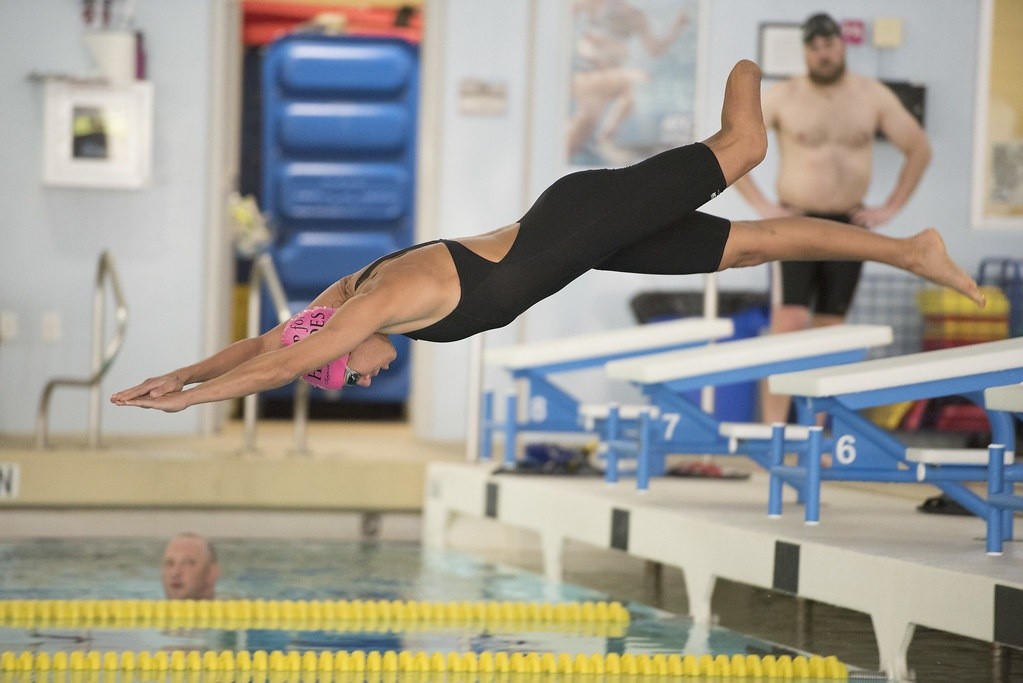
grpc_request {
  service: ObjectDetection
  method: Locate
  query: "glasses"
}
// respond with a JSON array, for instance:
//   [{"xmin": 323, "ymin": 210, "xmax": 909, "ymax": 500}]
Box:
[{"xmin": 344, "ymin": 363, "xmax": 360, "ymax": 386}]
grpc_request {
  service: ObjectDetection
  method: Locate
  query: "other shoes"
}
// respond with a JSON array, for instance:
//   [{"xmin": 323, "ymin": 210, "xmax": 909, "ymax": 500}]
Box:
[{"xmin": 918, "ymin": 495, "xmax": 971, "ymax": 518}]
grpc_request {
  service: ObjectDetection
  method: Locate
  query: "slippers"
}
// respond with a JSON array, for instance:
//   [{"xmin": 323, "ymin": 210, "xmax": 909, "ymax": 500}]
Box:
[{"xmin": 666, "ymin": 461, "xmax": 751, "ymax": 480}]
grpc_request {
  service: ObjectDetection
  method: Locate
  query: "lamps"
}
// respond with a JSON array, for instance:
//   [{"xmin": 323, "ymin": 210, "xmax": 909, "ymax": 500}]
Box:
[{"xmin": 870, "ymin": 15, "xmax": 901, "ymax": 48}]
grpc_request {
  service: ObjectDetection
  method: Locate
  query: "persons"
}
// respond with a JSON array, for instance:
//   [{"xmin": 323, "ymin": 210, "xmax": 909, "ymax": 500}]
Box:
[
  {"xmin": 110, "ymin": 60, "xmax": 987, "ymax": 413},
  {"xmin": 161, "ymin": 531, "xmax": 221, "ymax": 600},
  {"xmin": 732, "ymin": 12, "xmax": 932, "ymax": 467},
  {"xmin": 566, "ymin": 0, "xmax": 691, "ymax": 167}
]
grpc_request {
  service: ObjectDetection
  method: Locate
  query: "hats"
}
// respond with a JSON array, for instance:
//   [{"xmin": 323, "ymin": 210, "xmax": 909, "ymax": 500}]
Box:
[
  {"xmin": 801, "ymin": 14, "xmax": 840, "ymax": 42},
  {"xmin": 281, "ymin": 306, "xmax": 350, "ymax": 390}
]
